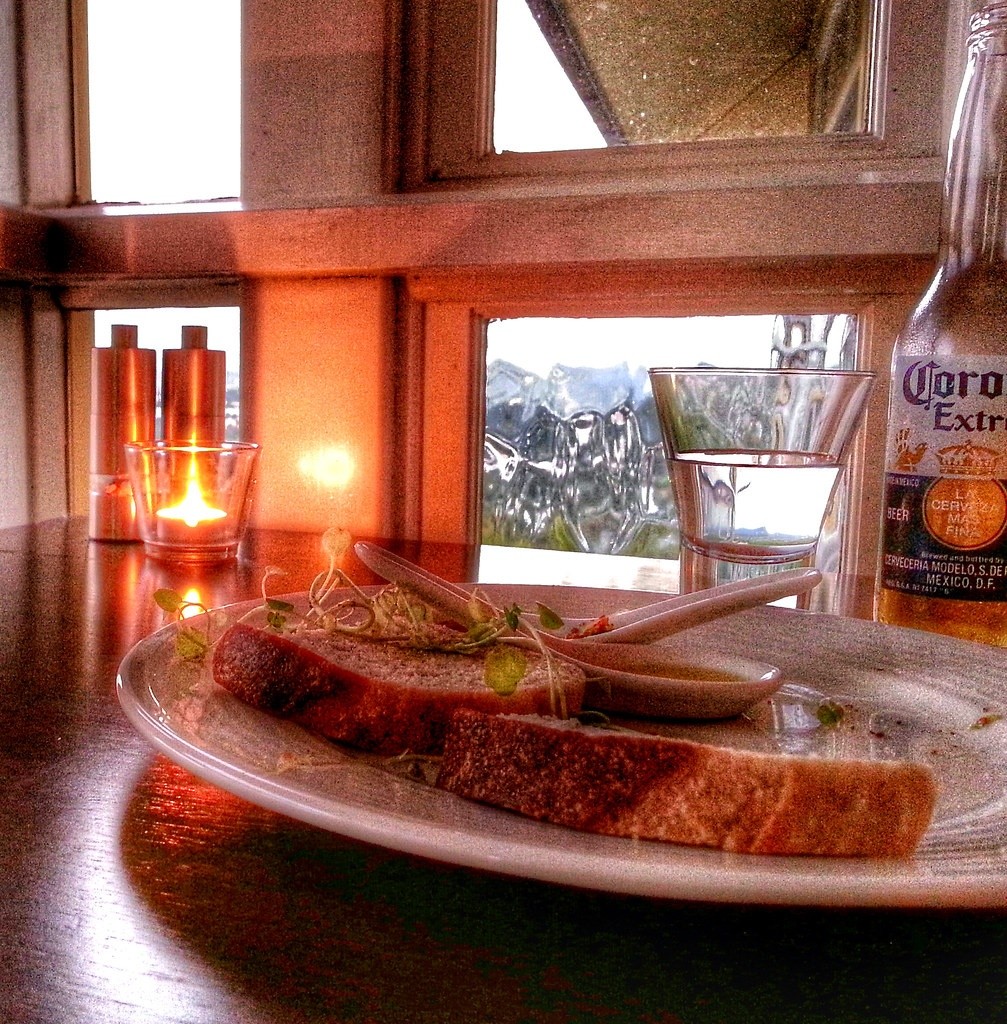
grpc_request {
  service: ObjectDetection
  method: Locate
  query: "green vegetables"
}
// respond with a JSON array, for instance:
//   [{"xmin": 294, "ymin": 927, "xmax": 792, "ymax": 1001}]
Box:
[{"xmin": 151, "ymin": 524, "xmax": 844, "ymax": 784}]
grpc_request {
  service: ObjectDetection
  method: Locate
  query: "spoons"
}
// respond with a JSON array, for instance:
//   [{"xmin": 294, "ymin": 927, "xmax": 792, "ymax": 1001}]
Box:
[
  {"xmin": 505, "ymin": 567, "xmax": 821, "ymax": 644},
  {"xmin": 358, "ymin": 540, "xmax": 785, "ymax": 720}
]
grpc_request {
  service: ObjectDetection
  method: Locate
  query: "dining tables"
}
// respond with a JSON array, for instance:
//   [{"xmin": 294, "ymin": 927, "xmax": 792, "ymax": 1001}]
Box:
[{"xmin": 0, "ymin": 517, "xmax": 1006, "ymax": 1024}]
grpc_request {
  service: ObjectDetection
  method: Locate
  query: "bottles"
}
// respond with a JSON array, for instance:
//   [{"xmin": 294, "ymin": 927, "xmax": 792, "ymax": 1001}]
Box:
[
  {"xmin": 162, "ymin": 323, "xmax": 224, "ymax": 497},
  {"xmin": 89, "ymin": 323, "xmax": 156, "ymax": 541},
  {"xmin": 875, "ymin": 0, "xmax": 1005, "ymax": 650}
]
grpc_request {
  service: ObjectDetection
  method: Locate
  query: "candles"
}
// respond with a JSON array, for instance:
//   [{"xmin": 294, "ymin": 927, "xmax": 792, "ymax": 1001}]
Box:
[{"xmin": 155, "ymin": 505, "xmax": 229, "ymax": 543}]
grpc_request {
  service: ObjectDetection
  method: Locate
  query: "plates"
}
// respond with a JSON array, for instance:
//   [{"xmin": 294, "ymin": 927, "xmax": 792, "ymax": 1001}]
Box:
[{"xmin": 114, "ymin": 583, "xmax": 1005, "ymax": 909}]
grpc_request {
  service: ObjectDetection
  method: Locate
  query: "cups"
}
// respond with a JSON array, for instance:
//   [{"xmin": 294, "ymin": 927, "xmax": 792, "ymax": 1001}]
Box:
[
  {"xmin": 650, "ymin": 366, "xmax": 877, "ymax": 564},
  {"xmin": 123, "ymin": 440, "xmax": 264, "ymax": 565}
]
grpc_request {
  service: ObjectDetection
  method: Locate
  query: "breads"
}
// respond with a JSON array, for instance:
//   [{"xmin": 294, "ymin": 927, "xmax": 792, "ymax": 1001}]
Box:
[
  {"xmin": 433, "ymin": 713, "xmax": 938, "ymax": 859},
  {"xmin": 210, "ymin": 622, "xmax": 586, "ymax": 755}
]
